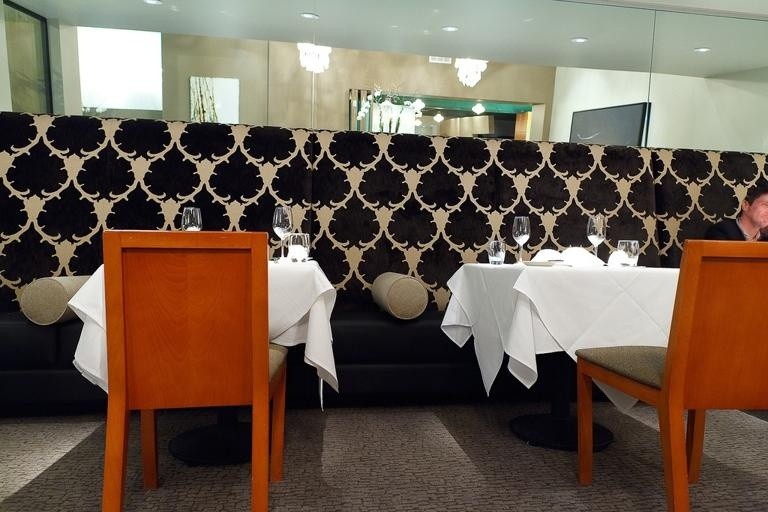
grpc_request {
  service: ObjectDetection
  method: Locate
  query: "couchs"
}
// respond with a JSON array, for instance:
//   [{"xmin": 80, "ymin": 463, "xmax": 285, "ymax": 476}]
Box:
[{"xmin": 20, "ymin": 113, "xmax": 743, "ymax": 416}]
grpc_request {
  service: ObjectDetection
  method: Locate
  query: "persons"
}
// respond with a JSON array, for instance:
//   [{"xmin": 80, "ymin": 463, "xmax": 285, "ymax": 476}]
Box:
[{"xmin": 704, "ymin": 181, "xmax": 768, "ymax": 241}]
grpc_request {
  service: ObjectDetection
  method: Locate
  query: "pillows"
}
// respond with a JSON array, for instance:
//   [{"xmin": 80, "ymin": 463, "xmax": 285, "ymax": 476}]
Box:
[
  {"xmin": 20, "ymin": 274, "xmax": 93, "ymax": 327},
  {"xmin": 371, "ymin": 272, "xmax": 430, "ymax": 322}
]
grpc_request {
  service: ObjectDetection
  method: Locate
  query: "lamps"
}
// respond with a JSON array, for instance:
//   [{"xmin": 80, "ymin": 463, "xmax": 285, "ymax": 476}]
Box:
[
  {"xmin": 451, "ymin": 57, "xmax": 489, "ymax": 90},
  {"xmin": 296, "ymin": 42, "xmax": 332, "ymax": 74}
]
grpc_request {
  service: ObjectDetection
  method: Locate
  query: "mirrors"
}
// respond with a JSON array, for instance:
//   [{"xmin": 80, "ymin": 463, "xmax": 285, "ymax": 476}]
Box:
[{"xmin": 0, "ymin": 0, "xmax": 768, "ymax": 156}]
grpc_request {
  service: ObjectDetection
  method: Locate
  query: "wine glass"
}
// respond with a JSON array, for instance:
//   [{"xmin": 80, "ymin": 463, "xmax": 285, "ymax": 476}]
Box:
[
  {"xmin": 511, "ymin": 217, "xmax": 533, "ymax": 261},
  {"xmin": 586, "ymin": 215, "xmax": 608, "ymax": 258},
  {"xmin": 272, "ymin": 205, "xmax": 292, "ymax": 262}
]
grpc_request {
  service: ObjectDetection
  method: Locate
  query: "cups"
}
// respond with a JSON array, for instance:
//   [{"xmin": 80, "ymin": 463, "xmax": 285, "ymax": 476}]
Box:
[
  {"xmin": 617, "ymin": 239, "xmax": 640, "ymax": 265},
  {"xmin": 183, "ymin": 207, "xmax": 201, "ymax": 232},
  {"xmin": 487, "ymin": 240, "xmax": 507, "ymax": 266},
  {"xmin": 288, "ymin": 231, "xmax": 310, "ymax": 262}
]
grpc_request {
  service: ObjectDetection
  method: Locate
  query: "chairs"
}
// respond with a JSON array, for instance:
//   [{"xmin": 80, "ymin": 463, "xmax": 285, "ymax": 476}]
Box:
[
  {"xmin": 574, "ymin": 239, "xmax": 768, "ymax": 512},
  {"xmin": 96, "ymin": 229, "xmax": 271, "ymax": 512}
]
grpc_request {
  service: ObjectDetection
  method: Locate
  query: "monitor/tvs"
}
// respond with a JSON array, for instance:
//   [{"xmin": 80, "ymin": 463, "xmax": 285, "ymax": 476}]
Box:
[{"xmin": 567, "ymin": 102, "xmax": 651, "ymax": 147}]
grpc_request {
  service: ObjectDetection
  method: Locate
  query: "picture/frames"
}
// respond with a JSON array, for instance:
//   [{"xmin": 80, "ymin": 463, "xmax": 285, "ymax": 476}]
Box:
[{"xmin": 2, "ymin": 0, "xmax": 54, "ymax": 115}]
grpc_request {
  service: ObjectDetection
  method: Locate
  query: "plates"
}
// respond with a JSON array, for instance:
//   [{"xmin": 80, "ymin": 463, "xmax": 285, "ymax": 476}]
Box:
[{"xmin": 526, "ymin": 260, "xmax": 555, "ymax": 265}]
